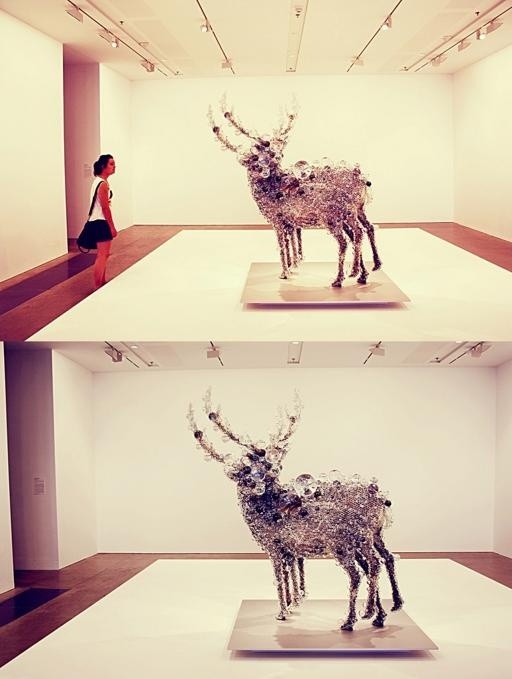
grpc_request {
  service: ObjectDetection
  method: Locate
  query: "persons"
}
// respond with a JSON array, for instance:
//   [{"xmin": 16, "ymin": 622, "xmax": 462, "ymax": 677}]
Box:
[{"xmin": 88, "ymin": 155, "xmax": 120, "ymax": 289}]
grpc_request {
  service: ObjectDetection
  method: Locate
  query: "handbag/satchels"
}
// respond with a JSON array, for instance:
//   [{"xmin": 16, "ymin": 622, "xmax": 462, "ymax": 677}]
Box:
[{"xmin": 76, "ymin": 221, "xmax": 97, "ymax": 254}]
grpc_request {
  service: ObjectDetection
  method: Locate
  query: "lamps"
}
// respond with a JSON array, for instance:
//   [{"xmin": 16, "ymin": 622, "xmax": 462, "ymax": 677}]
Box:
[
  {"xmin": 207, "ymin": 347, "xmax": 220, "ymax": 358},
  {"xmin": 199, "ymin": 19, "xmax": 209, "ymax": 33},
  {"xmin": 104, "ymin": 347, "xmax": 122, "ymax": 362},
  {"xmin": 431, "ymin": 21, "xmax": 503, "ymax": 67},
  {"xmin": 384, "ymin": 15, "xmax": 393, "ymax": 29},
  {"xmin": 471, "ymin": 345, "xmax": 492, "ymax": 358},
  {"xmin": 65, "ymin": 8, "xmax": 154, "ymax": 72},
  {"xmin": 369, "ymin": 344, "xmax": 385, "ymax": 356}
]
